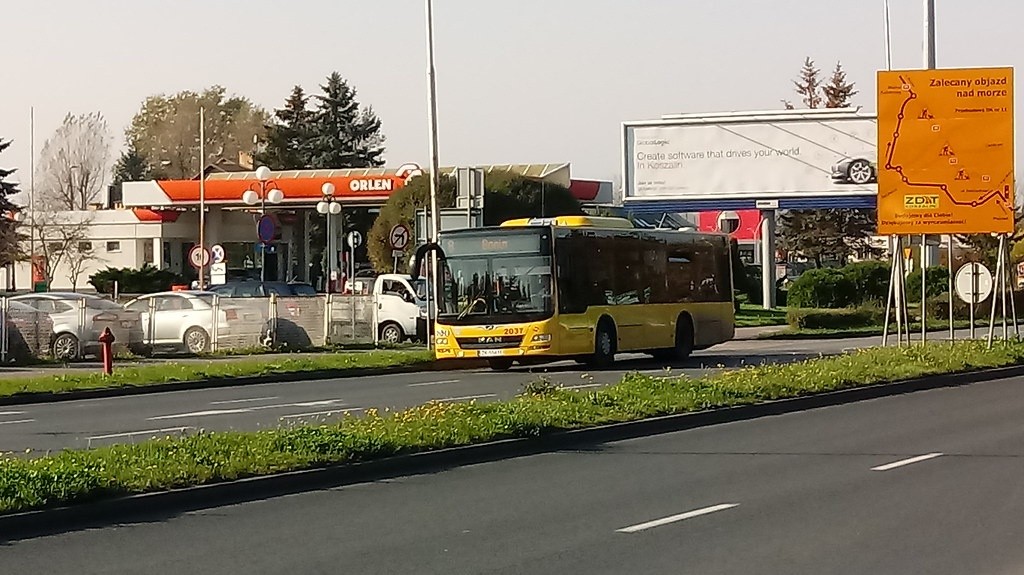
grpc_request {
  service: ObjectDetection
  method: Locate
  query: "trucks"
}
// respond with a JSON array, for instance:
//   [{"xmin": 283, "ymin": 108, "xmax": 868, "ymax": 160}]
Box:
[{"xmin": 321, "ymin": 273, "xmax": 439, "ymax": 348}]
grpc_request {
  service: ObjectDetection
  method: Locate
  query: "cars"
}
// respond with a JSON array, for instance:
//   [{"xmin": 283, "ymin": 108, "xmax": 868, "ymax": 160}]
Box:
[
  {"xmin": 344, "ymin": 269, "xmax": 378, "ymax": 296},
  {"xmin": 831, "ymin": 150, "xmax": 879, "ymax": 184},
  {"xmin": 775, "ymin": 274, "xmax": 803, "ymax": 308},
  {"xmin": 1, "ymin": 298, "xmax": 54, "ymax": 366},
  {"xmin": 122, "ymin": 292, "xmax": 264, "ymax": 355},
  {"xmin": 8, "ymin": 292, "xmax": 144, "ymax": 362}
]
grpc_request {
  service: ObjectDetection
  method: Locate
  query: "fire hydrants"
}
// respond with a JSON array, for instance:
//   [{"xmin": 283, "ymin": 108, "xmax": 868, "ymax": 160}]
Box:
[{"xmin": 99, "ymin": 327, "xmax": 114, "ymax": 376}]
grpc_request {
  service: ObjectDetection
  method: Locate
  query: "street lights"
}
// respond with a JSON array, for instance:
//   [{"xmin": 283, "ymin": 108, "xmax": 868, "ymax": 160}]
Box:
[
  {"xmin": 242, "ymin": 165, "xmax": 283, "ymax": 297},
  {"xmin": 317, "ymin": 183, "xmax": 341, "ymax": 291}
]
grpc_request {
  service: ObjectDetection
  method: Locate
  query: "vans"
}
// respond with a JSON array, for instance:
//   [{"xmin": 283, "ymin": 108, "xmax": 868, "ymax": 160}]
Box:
[{"xmin": 209, "ymin": 281, "xmax": 321, "ymax": 339}]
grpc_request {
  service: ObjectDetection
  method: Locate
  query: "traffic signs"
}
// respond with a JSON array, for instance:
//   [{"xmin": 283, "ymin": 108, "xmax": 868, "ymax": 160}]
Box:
[{"xmin": 874, "ymin": 68, "xmax": 1012, "ymax": 234}]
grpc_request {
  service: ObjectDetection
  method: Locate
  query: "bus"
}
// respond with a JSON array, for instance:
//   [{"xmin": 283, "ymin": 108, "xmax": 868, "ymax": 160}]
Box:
[{"xmin": 411, "ymin": 217, "xmax": 736, "ymax": 370}]
